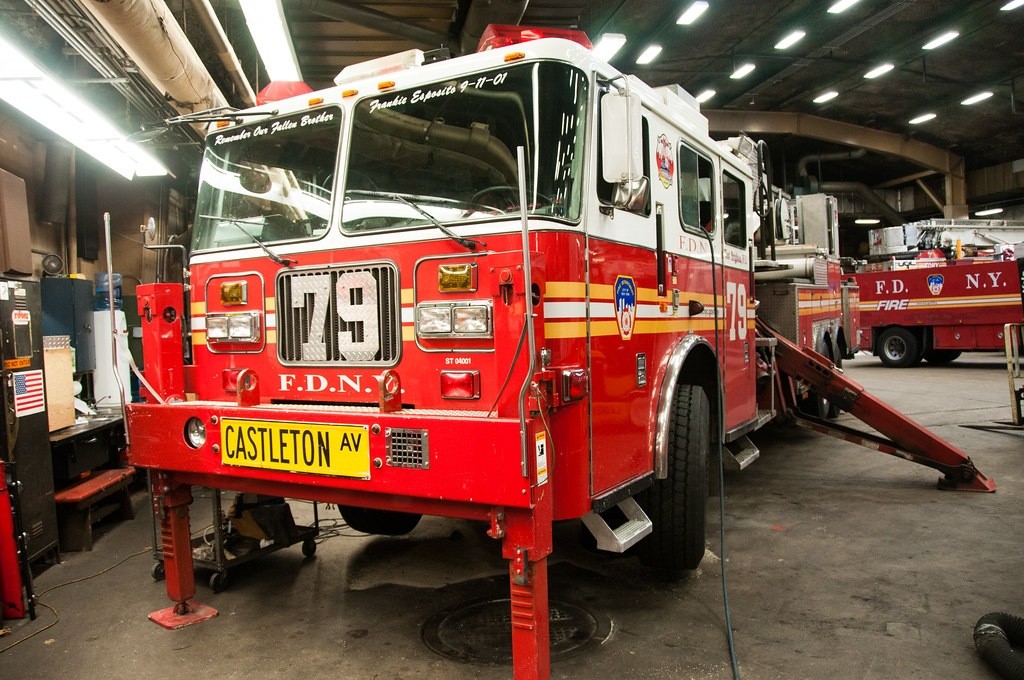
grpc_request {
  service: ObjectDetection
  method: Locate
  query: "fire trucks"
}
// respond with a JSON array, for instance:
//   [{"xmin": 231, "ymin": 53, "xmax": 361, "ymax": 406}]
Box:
[
  {"xmin": 116, "ymin": 22, "xmax": 1002, "ymax": 570},
  {"xmin": 840, "ymin": 215, "xmax": 1024, "ymax": 370}
]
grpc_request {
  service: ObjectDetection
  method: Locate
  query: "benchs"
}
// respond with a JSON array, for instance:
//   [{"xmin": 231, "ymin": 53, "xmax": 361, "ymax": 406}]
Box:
[{"xmin": 54, "ymin": 468, "xmax": 136, "ymax": 553}]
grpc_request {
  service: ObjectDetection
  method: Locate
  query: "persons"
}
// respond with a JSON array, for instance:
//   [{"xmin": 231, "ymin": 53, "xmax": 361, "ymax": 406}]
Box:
[{"xmin": 699, "ymin": 200, "xmax": 712, "ymax": 232}]
